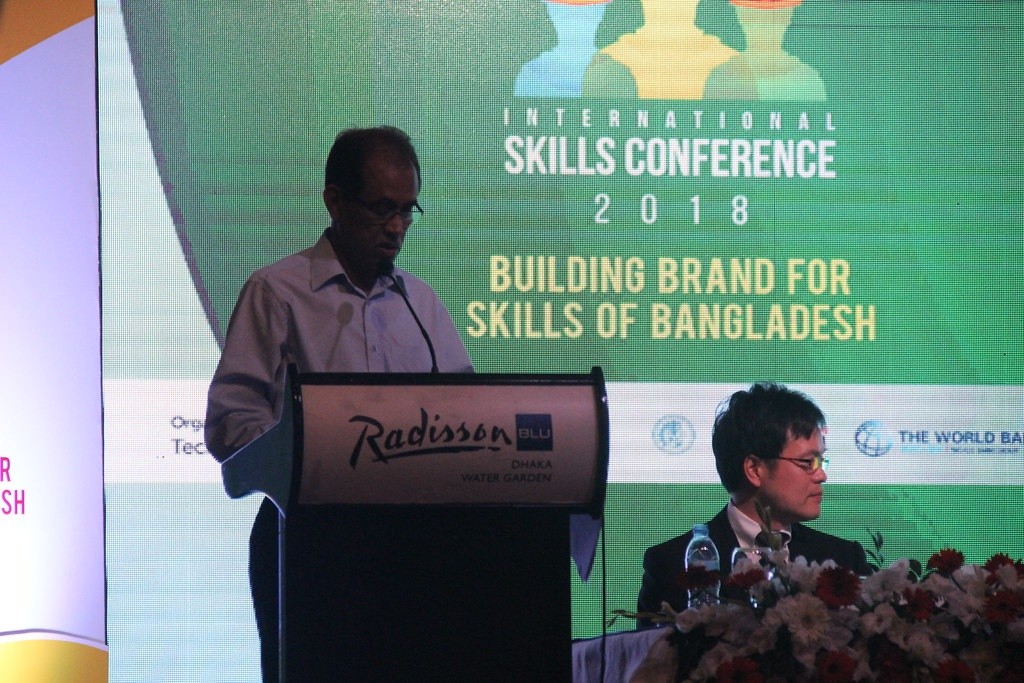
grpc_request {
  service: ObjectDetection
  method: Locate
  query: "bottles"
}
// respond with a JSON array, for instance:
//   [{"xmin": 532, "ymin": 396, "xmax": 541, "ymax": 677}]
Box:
[{"xmin": 685, "ymin": 524, "xmax": 721, "ymax": 608}]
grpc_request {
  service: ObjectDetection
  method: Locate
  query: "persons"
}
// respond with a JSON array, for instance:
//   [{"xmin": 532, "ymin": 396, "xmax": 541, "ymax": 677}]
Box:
[
  {"xmin": 637, "ymin": 380, "xmax": 867, "ymax": 632},
  {"xmin": 205, "ymin": 126, "xmax": 477, "ymax": 683}
]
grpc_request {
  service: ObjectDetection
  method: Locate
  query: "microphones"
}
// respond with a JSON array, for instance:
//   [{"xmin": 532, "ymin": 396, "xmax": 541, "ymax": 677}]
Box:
[{"xmin": 378, "ymin": 259, "xmax": 440, "ymax": 374}]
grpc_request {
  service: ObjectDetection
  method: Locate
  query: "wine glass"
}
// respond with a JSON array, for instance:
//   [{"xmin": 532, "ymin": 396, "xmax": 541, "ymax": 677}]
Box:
[{"xmin": 733, "ymin": 547, "xmax": 775, "ymax": 608}]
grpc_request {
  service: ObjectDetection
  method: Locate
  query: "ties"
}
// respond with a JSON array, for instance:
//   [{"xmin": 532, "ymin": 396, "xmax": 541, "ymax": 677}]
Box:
[{"xmin": 756, "ymin": 531, "xmax": 789, "ymax": 568}]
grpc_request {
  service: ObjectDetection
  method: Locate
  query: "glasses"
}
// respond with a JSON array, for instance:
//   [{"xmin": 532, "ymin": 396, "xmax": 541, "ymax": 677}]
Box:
[
  {"xmin": 760, "ymin": 456, "xmax": 830, "ymax": 472},
  {"xmin": 342, "ymin": 190, "xmax": 423, "ymax": 225}
]
